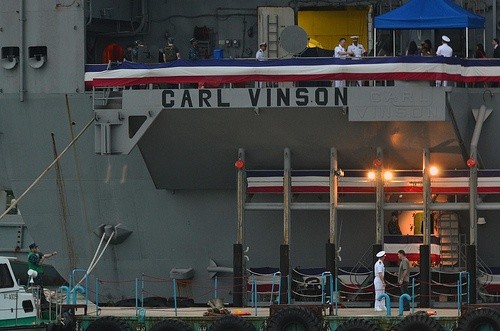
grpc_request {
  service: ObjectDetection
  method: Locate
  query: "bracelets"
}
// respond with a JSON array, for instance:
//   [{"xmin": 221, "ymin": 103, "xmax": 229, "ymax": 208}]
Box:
[{"xmin": 52, "ymin": 253, "xmax": 53, "ymax": 256}]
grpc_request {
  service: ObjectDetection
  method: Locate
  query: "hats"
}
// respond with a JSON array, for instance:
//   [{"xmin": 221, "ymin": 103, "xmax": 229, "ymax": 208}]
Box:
[
  {"xmin": 168, "ymin": 38, "xmax": 175, "ymax": 42},
  {"xmin": 442, "ymin": 35, "xmax": 450, "ymax": 42},
  {"xmin": 376, "ymin": 251, "xmax": 386, "ymax": 257},
  {"xmin": 350, "ymin": 36, "xmax": 359, "ymax": 40},
  {"xmin": 28, "ymin": 243, "xmax": 37, "ymax": 249},
  {"xmin": 134, "ymin": 40, "xmax": 141, "ymax": 44},
  {"xmin": 190, "ymin": 38, "xmax": 197, "ymax": 43},
  {"xmin": 259, "ymin": 42, "xmax": 266, "ymax": 46}
]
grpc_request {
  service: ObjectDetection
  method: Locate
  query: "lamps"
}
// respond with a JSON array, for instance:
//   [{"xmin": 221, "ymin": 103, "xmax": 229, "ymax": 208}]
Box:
[{"xmin": 477, "ymin": 217, "xmax": 486, "ymax": 225}]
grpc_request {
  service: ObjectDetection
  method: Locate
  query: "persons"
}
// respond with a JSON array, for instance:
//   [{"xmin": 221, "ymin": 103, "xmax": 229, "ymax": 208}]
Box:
[
  {"xmin": 28, "ymin": 243, "xmax": 57, "ymax": 304},
  {"xmin": 406, "ymin": 39, "xmax": 433, "ymax": 56},
  {"xmin": 398, "ymin": 251, "xmax": 410, "ymax": 311},
  {"xmin": 374, "ymin": 251, "xmax": 387, "ymax": 311},
  {"xmin": 492, "ymin": 39, "xmax": 500, "ymax": 87},
  {"xmin": 334, "ymin": 36, "xmax": 366, "ymax": 87},
  {"xmin": 256, "ymin": 43, "xmax": 267, "ymax": 88},
  {"xmin": 124, "ymin": 46, "xmax": 133, "ymax": 62},
  {"xmin": 436, "ymin": 36, "xmax": 452, "ymax": 86},
  {"xmin": 476, "ymin": 44, "xmax": 487, "ymax": 87},
  {"xmin": 189, "ymin": 39, "xmax": 200, "ymax": 88},
  {"xmin": 162, "ymin": 39, "xmax": 181, "ymax": 63}
]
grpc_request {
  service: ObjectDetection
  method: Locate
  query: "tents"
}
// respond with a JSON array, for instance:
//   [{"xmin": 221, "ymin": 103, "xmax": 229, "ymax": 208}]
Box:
[{"xmin": 374, "ymin": 0, "xmax": 485, "ymax": 88}]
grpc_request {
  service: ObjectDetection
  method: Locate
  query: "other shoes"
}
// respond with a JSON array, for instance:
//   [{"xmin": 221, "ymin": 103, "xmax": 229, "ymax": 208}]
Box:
[
  {"xmin": 375, "ymin": 309, "xmax": 383, "ymax": 312},
  {"xmin": 404, "ymin": 308, "xmax": 410, "ymax": 311}
]
